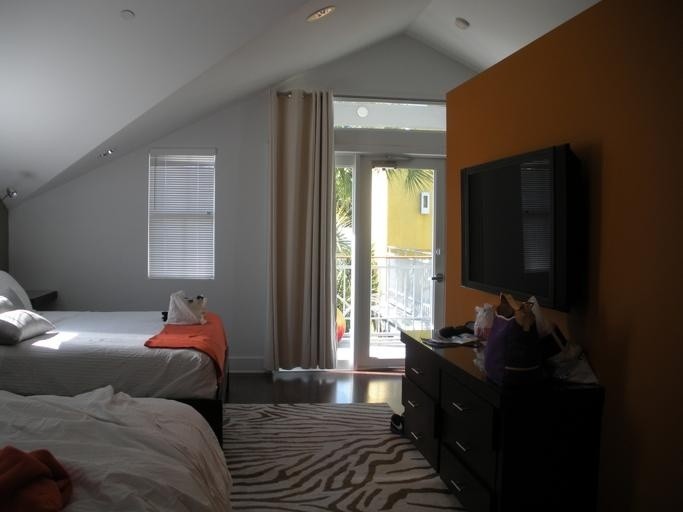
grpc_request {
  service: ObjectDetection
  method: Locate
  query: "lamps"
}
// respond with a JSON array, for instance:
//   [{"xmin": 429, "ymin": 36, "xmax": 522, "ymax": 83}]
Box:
[{"xmin": 0, "ymin": 186, "xmax": 18, "ymax": 201}]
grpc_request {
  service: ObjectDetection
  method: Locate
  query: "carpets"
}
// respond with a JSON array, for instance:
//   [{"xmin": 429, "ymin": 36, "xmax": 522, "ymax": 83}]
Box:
[{"xmin": 221, "ymin": 402, "xmax": 461, "ymax": 511}]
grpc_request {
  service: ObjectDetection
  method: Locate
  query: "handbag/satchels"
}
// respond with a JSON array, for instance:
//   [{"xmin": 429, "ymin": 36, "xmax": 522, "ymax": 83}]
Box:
[
  {"xmin": 164, "ymin": 290, "xmax": 206, "ymax": 326},
  {"xmin": 526, "ymin": 296, "xmax": 599, "ymax": 385},
  {"xmin": 481, "ymin": 291, "xmax": 549, "ymax": 384}
]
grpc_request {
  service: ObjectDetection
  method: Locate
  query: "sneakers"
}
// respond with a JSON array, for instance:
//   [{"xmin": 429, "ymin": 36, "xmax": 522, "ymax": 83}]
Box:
[{"xmin": 390, "ymin": 413, "xmax": 404, "ymax": 434}]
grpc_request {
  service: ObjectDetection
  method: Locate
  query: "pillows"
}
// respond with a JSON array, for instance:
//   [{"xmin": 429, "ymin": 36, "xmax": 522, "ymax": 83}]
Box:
[{"xmin": 0, "ymin": 270, "xmax": 57, "ymax": 344}]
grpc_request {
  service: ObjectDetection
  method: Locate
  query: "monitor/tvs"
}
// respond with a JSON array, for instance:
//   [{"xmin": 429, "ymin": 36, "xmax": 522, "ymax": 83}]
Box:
[{"xmin": 461, "ymin": 144, "xmax": 591, "ymax": 313}]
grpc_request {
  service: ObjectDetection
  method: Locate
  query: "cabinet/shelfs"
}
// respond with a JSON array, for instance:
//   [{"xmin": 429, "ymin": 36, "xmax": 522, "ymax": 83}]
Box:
[{"xmin": 399, "ymin": 329, "xmax": 604, "ymax": 511}]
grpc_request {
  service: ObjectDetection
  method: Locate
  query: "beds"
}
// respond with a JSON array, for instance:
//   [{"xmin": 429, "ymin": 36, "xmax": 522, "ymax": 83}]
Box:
[
  {"xmin": 0, "ymin": 393, "xmax": 223, "ymax": 511},
  {"xmin": 0, "ymin": 310, "xmax": 225, "ymax": 449}
]
